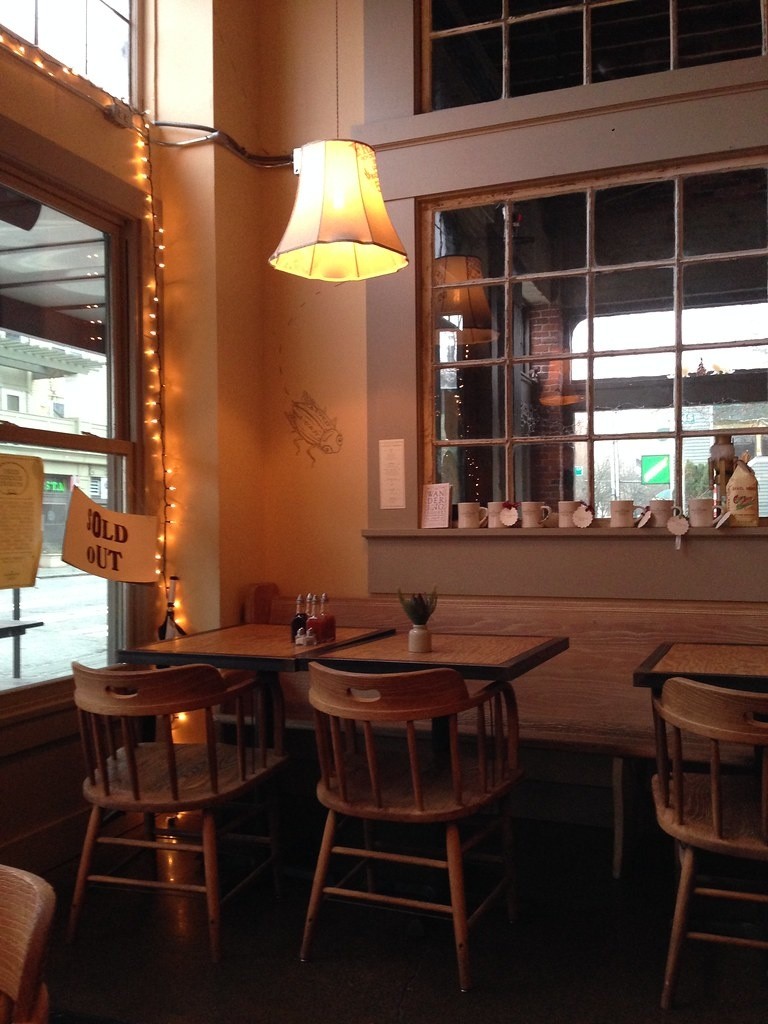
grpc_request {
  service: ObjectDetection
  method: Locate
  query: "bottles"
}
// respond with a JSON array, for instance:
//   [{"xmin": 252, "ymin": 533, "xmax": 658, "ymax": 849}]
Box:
[
  {"xmin": 289, "ymin": 591, "xmax": 337, "ymax": 646},
  {"xmin": 724, "ymin": 460, "xmax": 759, "ymax": 527}
]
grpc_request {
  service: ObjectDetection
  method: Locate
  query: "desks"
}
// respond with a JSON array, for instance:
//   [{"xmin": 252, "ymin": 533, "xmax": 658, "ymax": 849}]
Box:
[
  {"xmin": 631, "ymin": 637, "xmax": 768, "ymax": 889},
  {"xmin": 0, "ymin": 584, "xmax": 43, "ymax": 681},
  {"xmin": 297, "ymin": 630, "xmax": 570, "ymax": 880},
  {"xmin": 112, "ymin": 620, "xmax": 396, "ymax": 865}
]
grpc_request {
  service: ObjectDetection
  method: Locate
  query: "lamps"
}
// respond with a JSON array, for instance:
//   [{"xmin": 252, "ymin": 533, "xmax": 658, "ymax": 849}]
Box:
[
  {"xmin": 267, "ymin": 0, "xmax": 410, "ymax": 283},
  {"xmin": 541, "ymin": 350, "xmax": 584, "ymax": 404},
  {"xmin": 434, "ymin": 255, "xmax": 501, "ymax": 347}
]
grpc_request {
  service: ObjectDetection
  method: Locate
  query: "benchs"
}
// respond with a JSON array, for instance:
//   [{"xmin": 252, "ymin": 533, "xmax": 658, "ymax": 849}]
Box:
[{"xmin": 212, "ymin": 579, "xmax": 768, "ymax": 880}]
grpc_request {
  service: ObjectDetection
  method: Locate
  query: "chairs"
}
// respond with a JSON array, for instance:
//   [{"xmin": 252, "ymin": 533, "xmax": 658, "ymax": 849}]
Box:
[
  {"xmin": 298, "ymin": 661, "xmax": 525, "ymax": 994},
  {"xmin": 64, "ymin": 661, "xmax": 289, "ymax": 954},
  {"xmin": 650, "ymin": 676, "xmax": 768, "ymax": 1008},
  {"xmin": 0, "ymin": 862, "xmax": 57, "ymax": 1024}
]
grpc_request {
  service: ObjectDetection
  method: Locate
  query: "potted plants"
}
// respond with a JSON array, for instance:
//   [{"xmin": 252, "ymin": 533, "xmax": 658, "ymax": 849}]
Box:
[{"xmin": 397, "ymin": 583, "xmax": 439, "ymax": 653}]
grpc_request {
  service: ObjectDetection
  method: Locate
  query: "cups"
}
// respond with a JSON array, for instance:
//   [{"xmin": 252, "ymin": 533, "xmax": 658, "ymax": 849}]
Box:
[
  {"xmin": 687, "ymin": 499, "xmax": 724, "ymax": 528},
  {"xmin": 557, "ymin": 500, "xmax": 586, "ymax": 528},
  {"xmin": 521, "ymin": 501, "xmax": 551, "ymax": 528},
  {"xmin": 487, "ymin": 501, "xmax": 514, "ymax": 528},
  {"xmin": 648, "ymin": 500, "xmax": 682, "ymax": 528},
  {"xmin": 456, "ymin": 501, "xmax": 488, "ymax": 528},
  {"xmin": 610, "ymin": 500, "xmax": 646, "ymax": 528}
]
